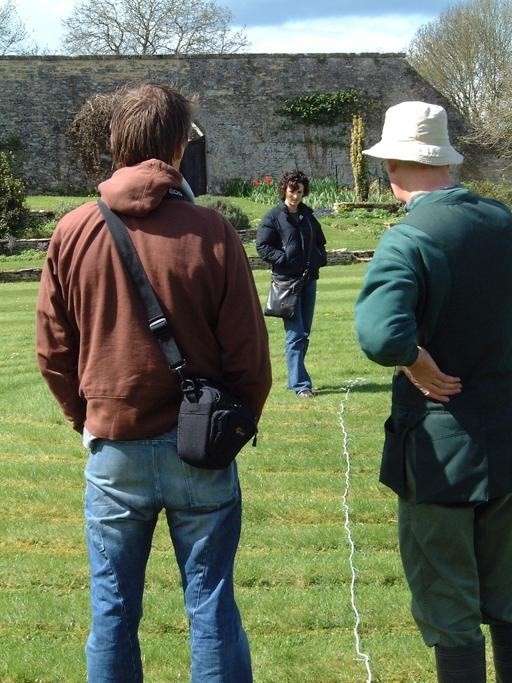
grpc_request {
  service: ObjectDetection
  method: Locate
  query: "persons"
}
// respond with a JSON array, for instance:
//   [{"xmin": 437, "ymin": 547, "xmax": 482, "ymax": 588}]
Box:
[
  {"xmin": 253, "ymin": 168, "xmax": 329, "ymax": 403},
  {"xmin": 346, "ymin": 100, "xmax": 510, "ymax": 683},
  {"xmin": 31, "ymin": 80, "xmax": 275, "ymax": 681}
]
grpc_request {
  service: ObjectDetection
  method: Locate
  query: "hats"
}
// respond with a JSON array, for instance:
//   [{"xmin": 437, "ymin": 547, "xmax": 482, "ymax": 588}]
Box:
[{"xmin": 362, "ymin": 101, "xmax": 465, "ymax": 167}]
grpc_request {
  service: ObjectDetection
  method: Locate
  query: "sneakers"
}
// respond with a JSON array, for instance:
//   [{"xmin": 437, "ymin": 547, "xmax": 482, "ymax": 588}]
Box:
[{"xmin": 297, "ymin": 389, "xmax": 312, "ymax": 399}]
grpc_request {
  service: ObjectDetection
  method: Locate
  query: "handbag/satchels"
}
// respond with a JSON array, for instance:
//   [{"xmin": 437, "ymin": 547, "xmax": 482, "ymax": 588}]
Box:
[
  {"xmin": 264, "ymin": 277, "xmax": 302, "ymax": 320},
  {"xmin": 177, "ymin": 378, "xmax": 257, "ymax": 470}
]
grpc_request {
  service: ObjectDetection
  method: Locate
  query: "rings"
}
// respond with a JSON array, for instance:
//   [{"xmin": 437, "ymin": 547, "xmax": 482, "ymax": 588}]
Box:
[{"xmin": 423, "ymin": 389, "xmax": 432, "ymax": 396}]
